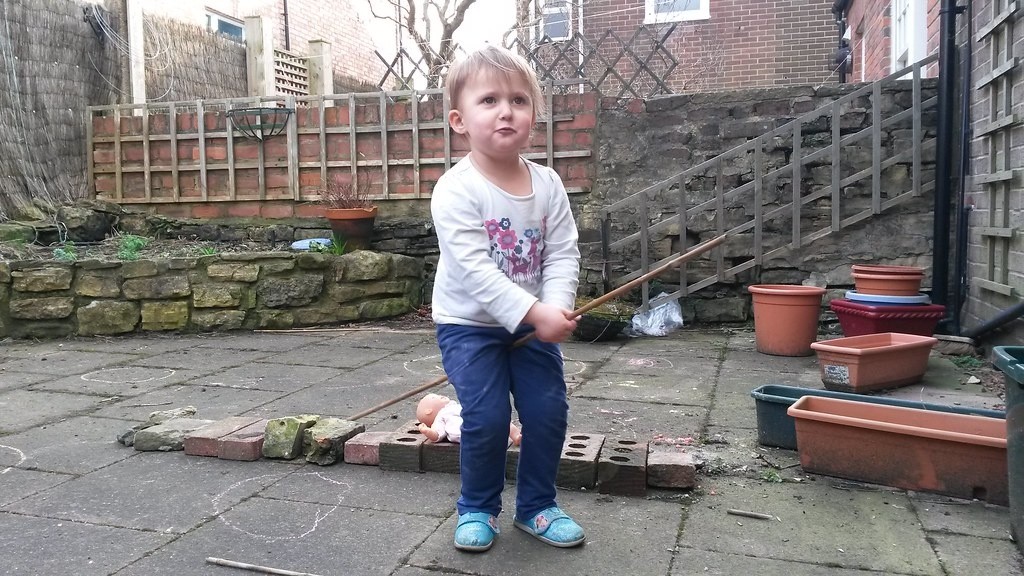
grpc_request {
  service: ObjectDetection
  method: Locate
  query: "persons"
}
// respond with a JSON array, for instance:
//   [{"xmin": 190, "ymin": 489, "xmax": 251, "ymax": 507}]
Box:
[
  {"xmin": 415, "ymin": 393, "xmax": 523, "ymax": 446},
  {"xmin": 429, "ymin": 43, "xmax": 586, "ymax": 552}
]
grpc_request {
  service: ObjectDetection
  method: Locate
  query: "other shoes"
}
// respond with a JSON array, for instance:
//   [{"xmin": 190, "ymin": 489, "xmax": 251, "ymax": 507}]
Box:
[
  {"xmin": 454, "ymin": 512, "xmax": 500, "ymax": 552},
  {"xmin": 513, "ymin": 507, "xmax": 587, "ymax": 547}
]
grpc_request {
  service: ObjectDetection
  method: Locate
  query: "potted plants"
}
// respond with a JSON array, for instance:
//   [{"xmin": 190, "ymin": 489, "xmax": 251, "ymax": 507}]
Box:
[
  {"xmin": 318, "ymin": 152, "xmax": 387, "ymax": 249},
  {"xmin": 571, "ymin": 295, "xmax": 642, "ymax": 341}
]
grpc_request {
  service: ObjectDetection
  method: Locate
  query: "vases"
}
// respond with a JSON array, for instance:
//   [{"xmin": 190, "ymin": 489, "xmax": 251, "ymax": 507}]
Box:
[
  {"xmin": 787, "ymin": 395, "xmax": 1010, "ymax": 507},
  {"xmin": 832, "ymin": 299, "xmax": 945, "ymax": 311},
  {"xmin": 811, "ymin": 332, "xmax": 939, "ymax": 395},
  {"xmin": 830, "ymin": 305, "xmax": 945, "ymax": 337},
  {"xmin": 852, "ymin": 264, "xmax": 926, "ymax": 275},
  {"xmin": 749, "ymin": 384, "xmax": 1005, "ymax": 451},
  {"xmin": 845, "ymin": 290, "xmax": 932, "ymax": 306},
  {"xmin": 852, "ymin": 272, "xmax": 925, "ymax": 296},
  {"xmin": 748, "ymin": 284, "xmax": 828, "ymax": 357},
  {"xmin": 991, "ymin": 344, "xmax": 1024, "ymax": 557}
]
